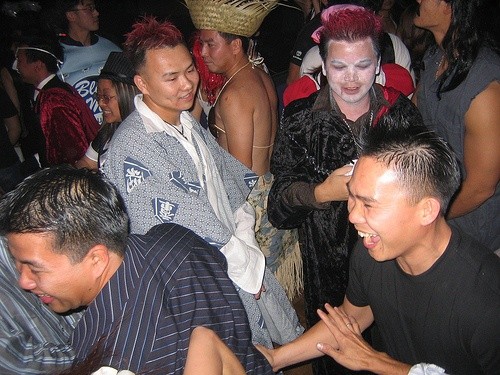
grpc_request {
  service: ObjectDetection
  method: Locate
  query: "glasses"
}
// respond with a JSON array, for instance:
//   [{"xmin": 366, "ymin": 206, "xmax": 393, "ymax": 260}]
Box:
[
  {"xmin": 68, "ymin": 4, "xmax": 98, "ymax": 14},
  {"xmin": 94, "ymin": 92, "xmax": 117, "ymax": 106}
]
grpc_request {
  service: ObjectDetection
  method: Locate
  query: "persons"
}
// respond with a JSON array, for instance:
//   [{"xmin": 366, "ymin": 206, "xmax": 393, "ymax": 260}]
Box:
[
  {"xmin": 16, "ymin": 33, "xmax": 101, "ymax": 164},
  {"xmin": 76, "ymin": 51, "xmax": 142, "ymax": 171},
  {"xmin": 103, "ymin": 16, "xmax": 304, "ymax": 349},
  {"xmin": 184, "ymin": 0, "xmax": 304, "ymax": 300},
  {"xmin": 57, "ymin": 0, "xmax": 123, "ymax": 126},
  {"xmin": 256, "ymin": 124, "xmax": 500, "ymax": 375},
  {"xmin": 0, "ymin": 10, "xmax": 49, "ymax": 193},
  {"xmin": 266, "ymin": 7, "xmax": 428, "ymax": 328},
  {"xmin": 0, "ymin": 162, "xmax": 273, "ymax": 375},
  {"xmin": 413, "ymin": 0, "xmax": 500, "ymax": 252},
  {"xmin": 283, "ymin": 0, "xmax": 434, "ymax": 108}
]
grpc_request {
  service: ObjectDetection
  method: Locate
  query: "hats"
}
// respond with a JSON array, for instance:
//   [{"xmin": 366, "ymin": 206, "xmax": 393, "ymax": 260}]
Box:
[
  {"xmin": 185, "ymin": 0, "xmax": 278, "ymax": 37},
  {"xmin": 99, "ymin": 50, "xmax": 136, "ymax": 89}
]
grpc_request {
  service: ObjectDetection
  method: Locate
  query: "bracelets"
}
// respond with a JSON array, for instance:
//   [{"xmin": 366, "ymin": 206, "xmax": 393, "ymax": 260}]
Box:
[{"xmin": 408, "ymin": 363, "xmax": 448, "ymax": 375}]
